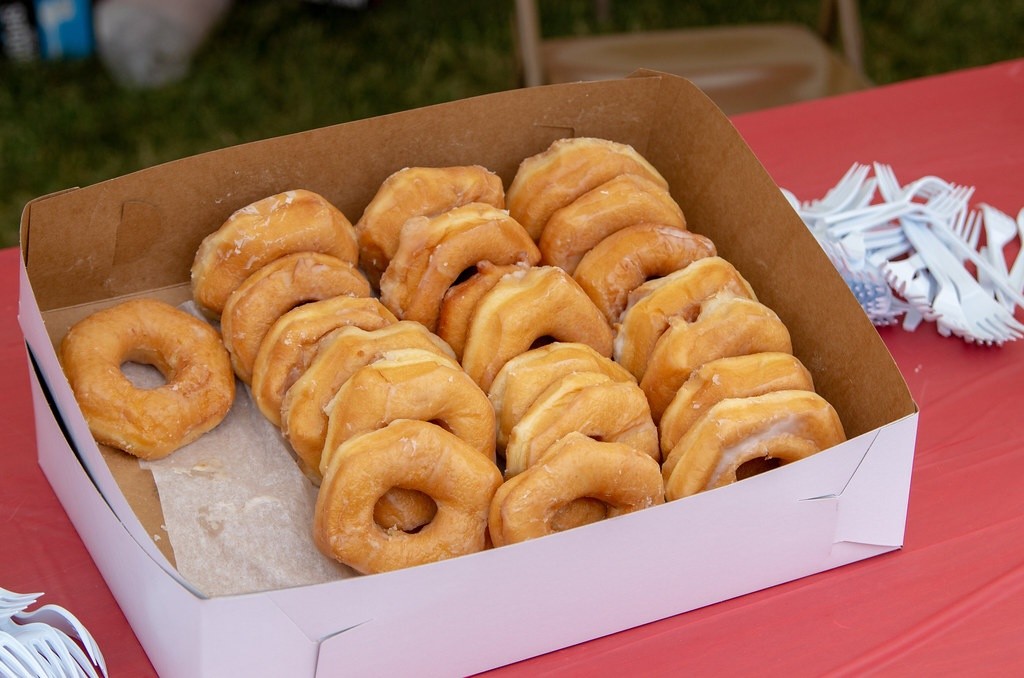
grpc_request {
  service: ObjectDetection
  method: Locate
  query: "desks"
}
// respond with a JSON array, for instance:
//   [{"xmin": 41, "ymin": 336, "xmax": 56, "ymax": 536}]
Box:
[{"xmin": 0, "ymin": 61, "xmax": 1023, "ymax": 677}]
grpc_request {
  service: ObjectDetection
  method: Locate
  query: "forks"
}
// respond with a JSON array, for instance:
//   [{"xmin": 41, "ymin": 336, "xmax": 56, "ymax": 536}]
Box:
[
  {"xmin": 0, "ymin": 586, "xmax": 109, "ymax": 678},
  {"xmin": 778, "ymin": 161, "xmax": 1024, "ymax": 347}
]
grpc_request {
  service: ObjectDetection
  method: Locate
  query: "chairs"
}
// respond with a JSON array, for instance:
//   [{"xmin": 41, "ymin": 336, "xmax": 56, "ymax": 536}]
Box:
[{"xmin": 513, "ymin": 0, "xmax": 874, "ymax": 115}]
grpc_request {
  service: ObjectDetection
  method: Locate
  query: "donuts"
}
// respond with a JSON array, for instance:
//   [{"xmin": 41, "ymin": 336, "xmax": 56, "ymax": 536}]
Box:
[
  {"xmin": 57, "ymin": 297, "xmax": 234, "ymax": 460},
  {"xmin": 188, "ymin": 138, "xmax": 851, "ymax": 575}
]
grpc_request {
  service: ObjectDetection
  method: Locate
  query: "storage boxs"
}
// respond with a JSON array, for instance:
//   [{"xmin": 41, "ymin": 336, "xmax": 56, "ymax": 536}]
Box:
[{"xmin": 18, "ymin": 68, "xmax": 918, "ymax": 678}]
